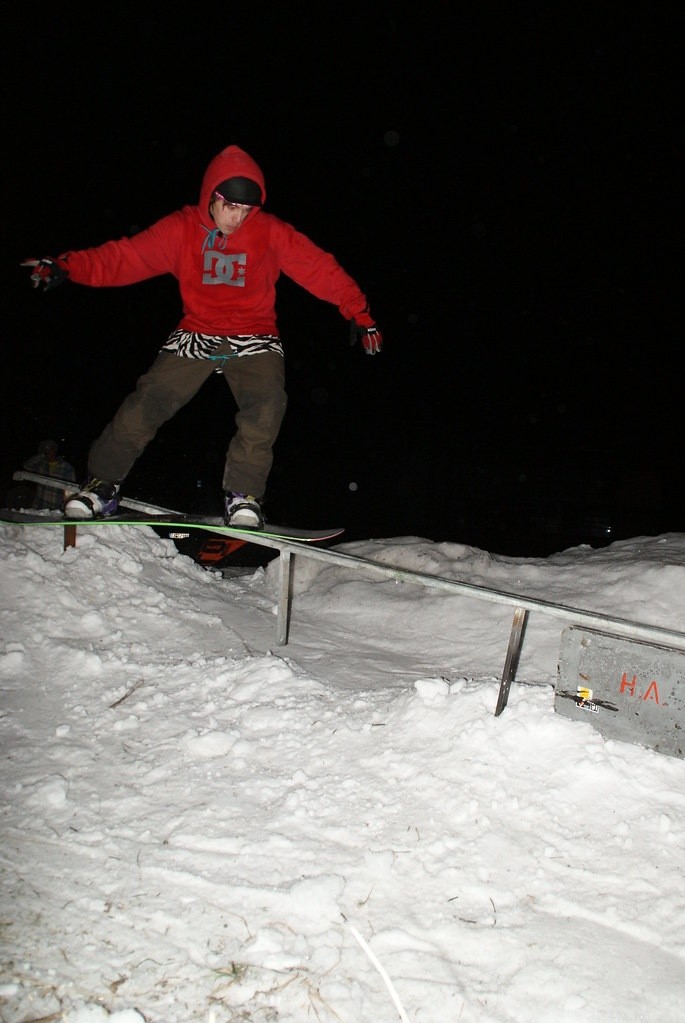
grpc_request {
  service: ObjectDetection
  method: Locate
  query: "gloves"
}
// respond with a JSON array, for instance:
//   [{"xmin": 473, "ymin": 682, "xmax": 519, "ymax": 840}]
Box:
[
  {"xmin": 362, "ymin": 326, "xmax": 383, "ymax": 355},
  {"xmin": 20, "ymin": 259, "xmax": 60, "ymax": 290}
]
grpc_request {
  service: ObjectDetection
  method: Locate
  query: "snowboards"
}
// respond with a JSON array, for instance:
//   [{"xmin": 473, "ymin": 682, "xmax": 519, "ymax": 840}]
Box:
[{"xmin": 0, "ymin": 509, "xmax": 343, "ymax": 542}]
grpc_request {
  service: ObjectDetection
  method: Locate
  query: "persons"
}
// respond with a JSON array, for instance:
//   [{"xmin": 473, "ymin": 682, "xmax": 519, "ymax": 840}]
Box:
[
  {"xmin": 19, "ymin": 144, "xmax": 382, "ymax": 530},
  {"xmin": 21, "ymin": 439, "xmax": 77, "ymax": 512}
]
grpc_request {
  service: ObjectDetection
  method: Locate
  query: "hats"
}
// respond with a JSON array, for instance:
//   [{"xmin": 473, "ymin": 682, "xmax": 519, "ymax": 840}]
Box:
[{"xmin": 216, "ymin": 177, "xmax": 262, "ymax": 206}]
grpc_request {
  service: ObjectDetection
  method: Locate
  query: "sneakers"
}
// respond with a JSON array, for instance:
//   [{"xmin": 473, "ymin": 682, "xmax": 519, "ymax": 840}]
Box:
[
  {"xmin": 224, "ymin": 492, "xmax": 264, "ymax": 531},
  {"xmin": 64, "ymin": 477, "xmax": 123, "ymax": 519}
]
